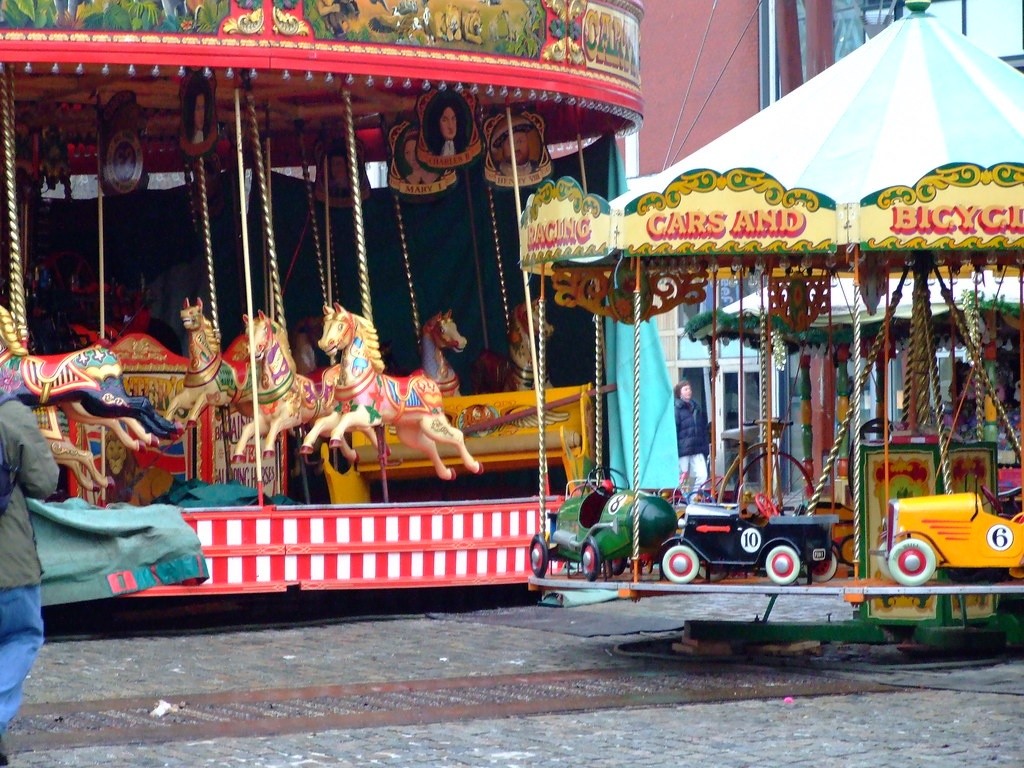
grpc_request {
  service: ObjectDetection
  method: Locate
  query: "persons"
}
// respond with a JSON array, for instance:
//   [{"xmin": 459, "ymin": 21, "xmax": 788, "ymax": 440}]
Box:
[
  {"xmin": 0, "ymin": 391, "xmax": 59, "ymax": 767},
  {"xmin": 672, "ymin": 382, "xmax": 708, "ymax": 501}
]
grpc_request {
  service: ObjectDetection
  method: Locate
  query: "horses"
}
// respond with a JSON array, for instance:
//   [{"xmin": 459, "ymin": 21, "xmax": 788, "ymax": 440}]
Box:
[{"xmin": 0, "ymin": 296, "xmax": 556, "ymax": 493}]
